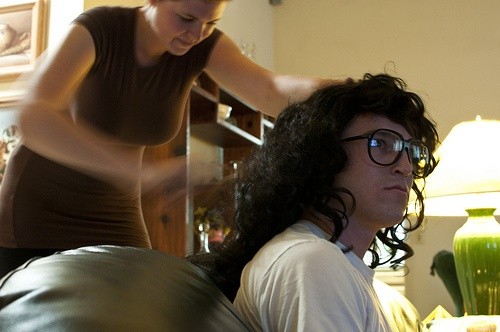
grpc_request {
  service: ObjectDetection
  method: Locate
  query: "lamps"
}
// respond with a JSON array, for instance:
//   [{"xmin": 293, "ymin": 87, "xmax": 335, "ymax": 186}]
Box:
[{"xmin": 405, "ymin": 119, "xmax": 500, "ymax": 315}]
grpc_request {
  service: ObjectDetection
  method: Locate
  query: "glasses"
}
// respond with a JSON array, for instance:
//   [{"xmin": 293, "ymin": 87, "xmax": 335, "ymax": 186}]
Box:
[{"xmin": 343, "ymin": 128, "xmax": 431, "ymax": 179}]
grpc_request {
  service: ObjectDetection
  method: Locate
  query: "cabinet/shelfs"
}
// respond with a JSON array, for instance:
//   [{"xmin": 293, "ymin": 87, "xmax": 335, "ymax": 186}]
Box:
[{"xmin": 143, "ymin": 74, "xmax": 275, "ymax": 260}]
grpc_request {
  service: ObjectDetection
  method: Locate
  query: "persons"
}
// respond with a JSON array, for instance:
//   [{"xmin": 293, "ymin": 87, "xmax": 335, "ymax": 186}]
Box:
[
  {"xmin": 0, "ymin": 0, "xmax": 336, "ymax": 276},
  {"xmin": 204, "ymin": 67, "xmax": 442, "ymax": 332}
]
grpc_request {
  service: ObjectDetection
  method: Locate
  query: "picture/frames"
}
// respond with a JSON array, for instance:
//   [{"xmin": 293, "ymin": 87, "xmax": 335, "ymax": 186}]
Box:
[{"xmin": 0, "ymin": 0, "xmax": 51, "ymax": 108}]
